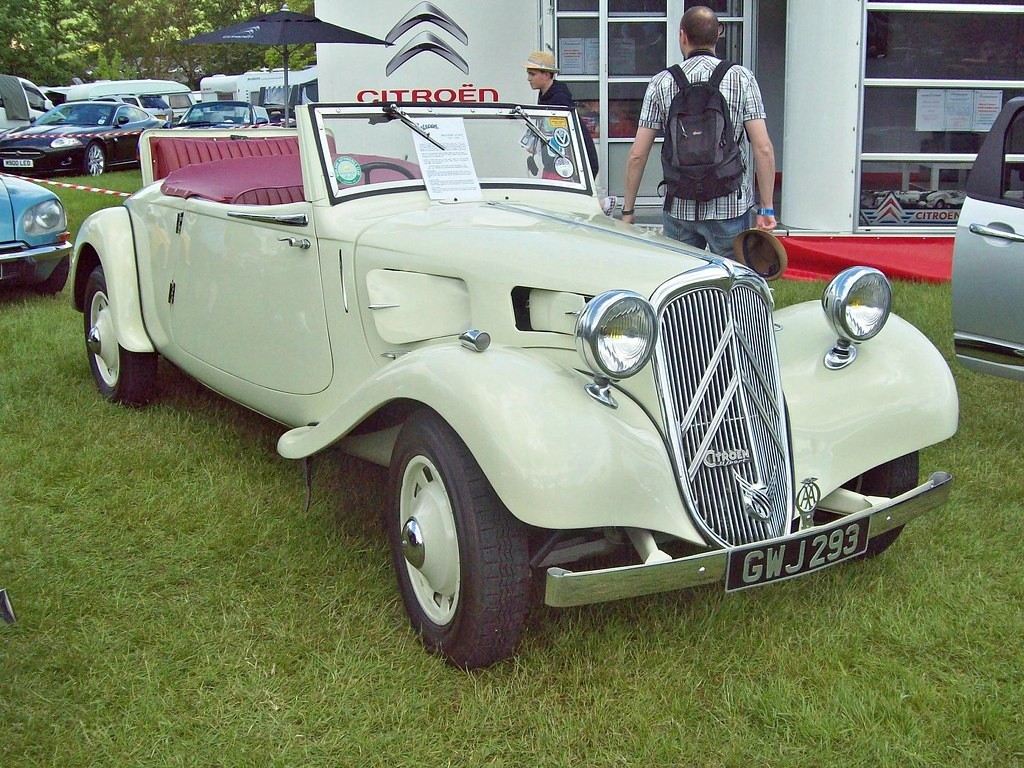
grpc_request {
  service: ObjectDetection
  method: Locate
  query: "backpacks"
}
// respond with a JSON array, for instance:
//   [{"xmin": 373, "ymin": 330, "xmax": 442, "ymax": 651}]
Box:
[{"xmin": 657, "ymin": 60, "xmax": 747, "ymax": 202}]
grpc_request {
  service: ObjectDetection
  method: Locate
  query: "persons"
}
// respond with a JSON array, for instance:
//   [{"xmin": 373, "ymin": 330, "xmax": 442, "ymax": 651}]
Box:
[
  {"xmin": 621, "ymin": 6, "xmax": 777, "ymax": 265},
  {"xmin": 521, "ymin": 50, "xmax": 618, "ymax": 218}
]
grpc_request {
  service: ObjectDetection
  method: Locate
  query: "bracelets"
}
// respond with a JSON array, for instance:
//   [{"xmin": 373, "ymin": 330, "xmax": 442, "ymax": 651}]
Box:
[
  {"xmin": 757, "ymin": 207, "xmax": 775, "ymax": 215},
  {"xmin": 622, "ymin": 204, "xmax": 635, "ymax": 216}
]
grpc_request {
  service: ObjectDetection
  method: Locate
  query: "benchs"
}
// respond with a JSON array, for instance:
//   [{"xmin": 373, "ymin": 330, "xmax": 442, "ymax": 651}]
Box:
[
  {"xmin": 156, "ymin": 133, "xmax": 336, "ymax": 179},
  {"xmin": 161, "ymin": 153, "xmax": 422, "ymax": 206}
]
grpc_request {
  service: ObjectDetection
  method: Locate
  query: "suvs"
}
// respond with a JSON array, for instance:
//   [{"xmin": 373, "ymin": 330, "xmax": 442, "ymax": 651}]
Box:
[{"xmin": 89, "ymin": 93, "xmax": 174, "ymax": 122}]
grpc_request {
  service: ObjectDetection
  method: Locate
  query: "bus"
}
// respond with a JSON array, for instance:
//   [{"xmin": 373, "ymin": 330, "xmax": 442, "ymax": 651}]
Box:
[{"xmin": 43, "ymin": 76, "xmax": 200, "ymax": 124}]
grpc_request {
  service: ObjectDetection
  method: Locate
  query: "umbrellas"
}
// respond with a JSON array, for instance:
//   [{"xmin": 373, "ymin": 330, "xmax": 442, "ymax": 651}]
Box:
[{"xmin": 177, "ymin": 4, "xmax": 396, "ymax": 128}]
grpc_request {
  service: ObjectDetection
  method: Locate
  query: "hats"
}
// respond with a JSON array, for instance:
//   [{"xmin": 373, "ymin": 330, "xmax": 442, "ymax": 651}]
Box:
[
  {"xmin": 734, "ymin": 228, "xmax": 788, "ymax": 282},
  {"xmin": 520, "ymin": 51, "xmax": 559, "ymax": 73}
]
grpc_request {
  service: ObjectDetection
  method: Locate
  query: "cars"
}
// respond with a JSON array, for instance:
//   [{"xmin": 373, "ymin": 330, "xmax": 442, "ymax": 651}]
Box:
[
  {"xmin": 0, "ymin": 175, "xmax": 74, "ymax": 296},
  {"xmin": 162, "ymin": 100, "xmax": 269, "ymax": 130},
  {"xmin": 257, "ymin": 103, "xmax": 295, "ymax": 124},
  {"xmin": 0, "ymin": 100, "xmax": 172, "ymax": 177},
  {"xmin": 66, "ymin": 102, "xmax": 961, "ymax": 670}
]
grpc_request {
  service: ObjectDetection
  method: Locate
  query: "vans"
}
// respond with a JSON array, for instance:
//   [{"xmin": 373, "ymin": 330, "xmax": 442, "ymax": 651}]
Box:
[{"xmin": 0, "ymin": 74, "xmax": 68, "ymax": 130}]
